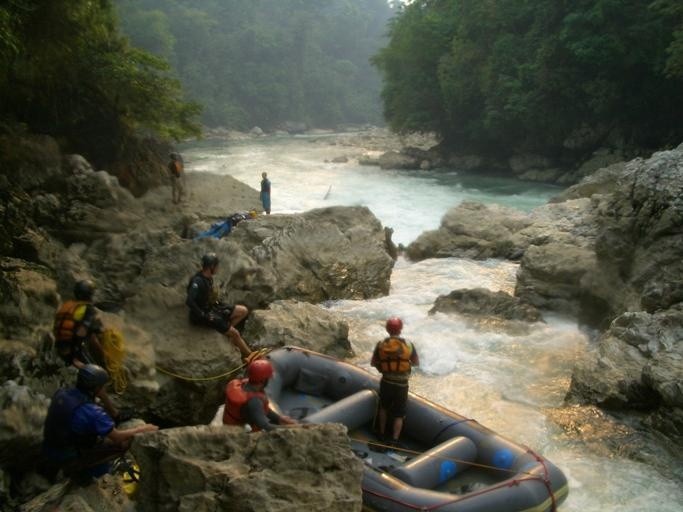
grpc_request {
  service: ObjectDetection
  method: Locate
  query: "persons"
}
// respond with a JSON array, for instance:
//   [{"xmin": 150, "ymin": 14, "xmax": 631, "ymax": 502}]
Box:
[
  {"xmin": 184, "ymin": 251, "xmax": 252, "ymax": 356},
  {"xmin": 369, "ymin": 316, "xmax": 420, "ymax": 448},
  {"xmin": 222, "ymin": 359, "xmax": 296, "ymax": 432},
  {"xmin": 168, "ymin": 152, "xmax": 184, "ymax": 203},
  {"xmin": 195, "ymin": 209, "xmax": 257, "ymax": 241},
  {"xmin": 34, "ymin": 362, "xmax": 158, "ymax": 488},
  {"xmin": 259, "ymin": 172, "xmax": 273, "ymax": 214},
  {"xmin": 52, "ymin": 279, "xmax": 119, "ymax": 418}
]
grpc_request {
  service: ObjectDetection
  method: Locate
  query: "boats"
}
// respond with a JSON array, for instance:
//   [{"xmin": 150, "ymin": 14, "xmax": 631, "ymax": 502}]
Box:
[{"xmin": 256, "ymin": 346, "xmax": 569, "ymax": 511}]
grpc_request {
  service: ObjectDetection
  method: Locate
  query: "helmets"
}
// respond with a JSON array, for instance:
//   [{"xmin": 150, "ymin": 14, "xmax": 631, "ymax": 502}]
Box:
[
  {"xmin": 75, "ymin": 365, "xmax": 109, "ymax": 397},
  {"xmin": 248, "ymin": 359, "xmax": 274, "ymax": 382},
  {"xmin": 386, "ymin": 317, "xmax": 402, "ymax": 334},
  {"xmin": 75, "ymin": 280, "xmax": 96, "ymax": 301},
  {"xmin": 202, "ymin": 252, "xmax": 218, "ymax": 266}
]
